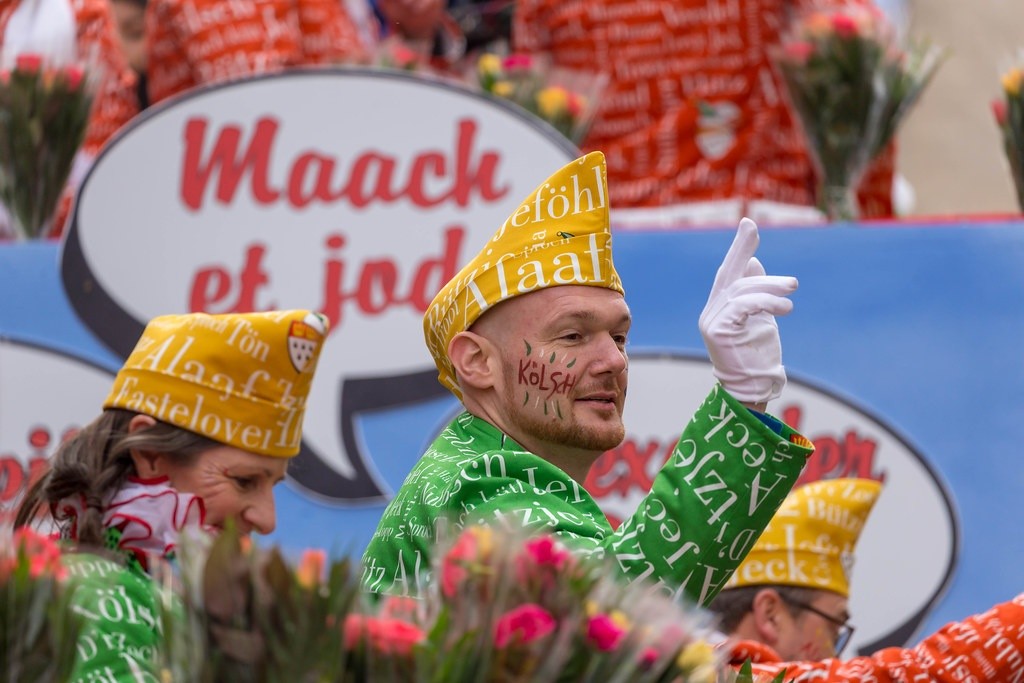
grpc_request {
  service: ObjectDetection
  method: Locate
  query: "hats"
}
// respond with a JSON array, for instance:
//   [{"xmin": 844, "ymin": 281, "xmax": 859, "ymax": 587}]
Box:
[
  {"xmin": 422, "ymin": 150, "xmax": 626, "ymax": 408},
  {"xmin": 102, "ymin": 309, "xmax": 330, "ymax": 459},
  {"xmin": 719, "ymin": 478, "xmax": 883, "ymax": 599}
]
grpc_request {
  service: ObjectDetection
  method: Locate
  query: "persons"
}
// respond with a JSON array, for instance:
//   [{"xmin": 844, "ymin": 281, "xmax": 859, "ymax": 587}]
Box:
[
  {"xmin": 355, "ymin": 151, "xmax": 817, "ymax": 683},
  {"xmin": 0, "ymin": 0, "xmax": 919, "ymax": 226},
  {"xmin": 706, "ymin": 479, "xmax": 1024, "ymax": 683},
  {"xmin": 1, "ymin": 308, "xmax": 329, "ymax": 683}
]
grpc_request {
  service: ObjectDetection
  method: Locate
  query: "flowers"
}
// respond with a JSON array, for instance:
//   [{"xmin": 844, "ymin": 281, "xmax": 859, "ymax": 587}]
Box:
[
  {"xmin": 477, "ymin": 50, "xmax": 608, "ymax": 145},
  {"xmin": 2, "ymin": 509, "xmax": 802, "ymax": 683},
  {"xmin": 991, "ymin": 68, "xmax": 1023, "ymax": 216},
  {"xmin": 770, "ymin": 0, "xmax": 954, "ymax": 226},
  {"xmin": 1, "ymin": 51, "xmax": 100, "ymax": 245}
]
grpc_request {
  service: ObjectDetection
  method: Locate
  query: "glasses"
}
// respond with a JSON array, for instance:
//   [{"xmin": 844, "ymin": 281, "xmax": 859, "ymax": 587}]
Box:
[{"xmin": 777, "ymin": 593, "xmax": 854, "ymax": 658}]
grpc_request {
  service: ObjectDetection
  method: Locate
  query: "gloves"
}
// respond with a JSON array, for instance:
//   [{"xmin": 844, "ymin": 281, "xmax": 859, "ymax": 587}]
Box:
[{"xmin": 697, "ymin": 216, "xmax": 798, "ymax": 406}]
